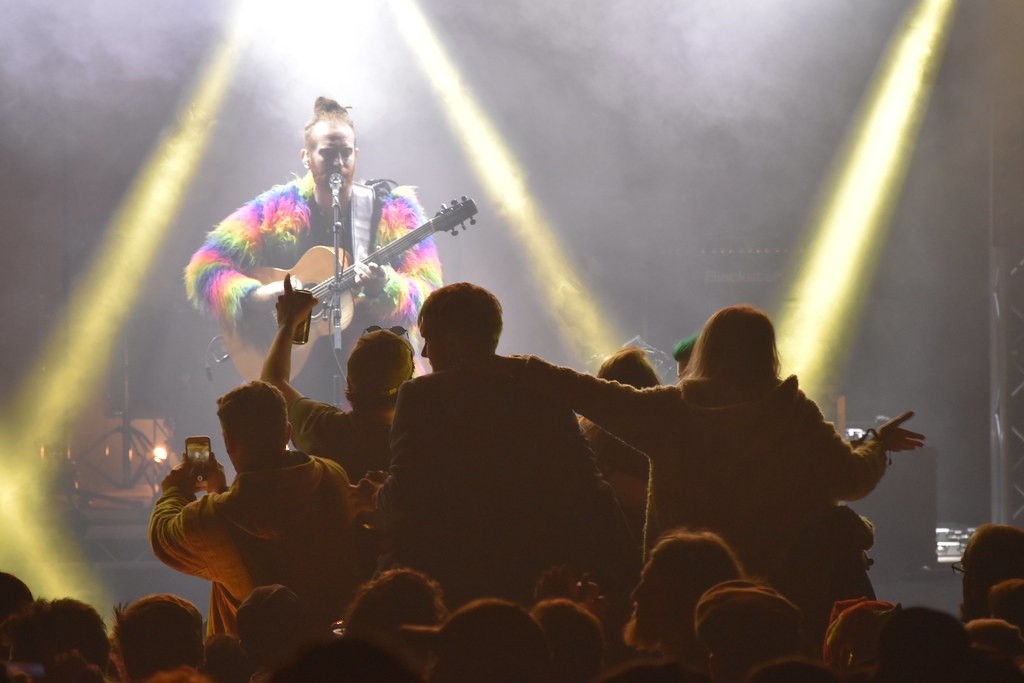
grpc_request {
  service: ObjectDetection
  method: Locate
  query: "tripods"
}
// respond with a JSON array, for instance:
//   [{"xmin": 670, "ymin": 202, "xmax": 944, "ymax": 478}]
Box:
[{"xmin": 52, "ymin": 331, "xmax": 173, "ymax": 507}]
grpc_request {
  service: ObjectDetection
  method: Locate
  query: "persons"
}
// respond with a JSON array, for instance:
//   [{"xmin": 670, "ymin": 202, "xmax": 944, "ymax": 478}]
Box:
[
  {"xmin": 491, "ymin": 306, "xmax": 927, "ymax": 604},
  {"xmin": 184, "ymin": 97, "xmax": 443, "ymax": 409},
  {"xmin": 148, "ymin": 379, "xmax": 355, "ymax": 639},
  {"xmin": 349, "ymin": 283, "xmax": 643, "ymax": 607},
  {"xmin": 263, "ymin": 273, "xmax": 414, "ymax": 482},
  {"xmin": 0, "ymin": 524, "xmax": 1024, "ymax": 683}
]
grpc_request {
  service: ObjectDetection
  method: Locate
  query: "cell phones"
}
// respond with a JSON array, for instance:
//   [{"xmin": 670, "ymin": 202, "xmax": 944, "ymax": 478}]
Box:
[{"xmin": 185, "ymin": 435, "xmax": 211, "ymax": 490}]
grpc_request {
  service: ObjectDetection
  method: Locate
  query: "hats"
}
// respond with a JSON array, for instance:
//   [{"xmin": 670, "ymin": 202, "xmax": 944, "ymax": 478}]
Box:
[
  {"xmin": 696, "ymin": 579, "xmax": 804, "ymax": 650},
  {"xmin": 346, "ymin": 330, "xmax": 414, "ymax": 396}
]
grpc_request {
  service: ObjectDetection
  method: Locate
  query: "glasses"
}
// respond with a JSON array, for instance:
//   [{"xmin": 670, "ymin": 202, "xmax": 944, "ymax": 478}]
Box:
[
  {"xmin": 951, "ymin": 562, "xmax": 967, "ymax": 575},
  {"xmin": 361, "ymin": 325, "xmax": 409, "ymax": 341}
]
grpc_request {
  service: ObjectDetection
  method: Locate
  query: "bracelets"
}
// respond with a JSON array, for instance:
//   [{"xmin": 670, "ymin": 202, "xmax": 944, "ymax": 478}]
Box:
[{"xmin": 852, "ymin": 428, "xmax": 892, "ymax": 467}]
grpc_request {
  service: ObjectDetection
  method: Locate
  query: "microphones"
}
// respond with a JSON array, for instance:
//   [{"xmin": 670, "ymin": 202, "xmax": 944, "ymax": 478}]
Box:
[{"xmin": 329, "ymin": 173, "xmax": 344, "ymax": 197}]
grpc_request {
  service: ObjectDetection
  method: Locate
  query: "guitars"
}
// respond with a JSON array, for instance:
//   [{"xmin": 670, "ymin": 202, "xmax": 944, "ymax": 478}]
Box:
[{"xmin": 218, "ymin": 193, "xmax": 480, "ymax": 388}]
